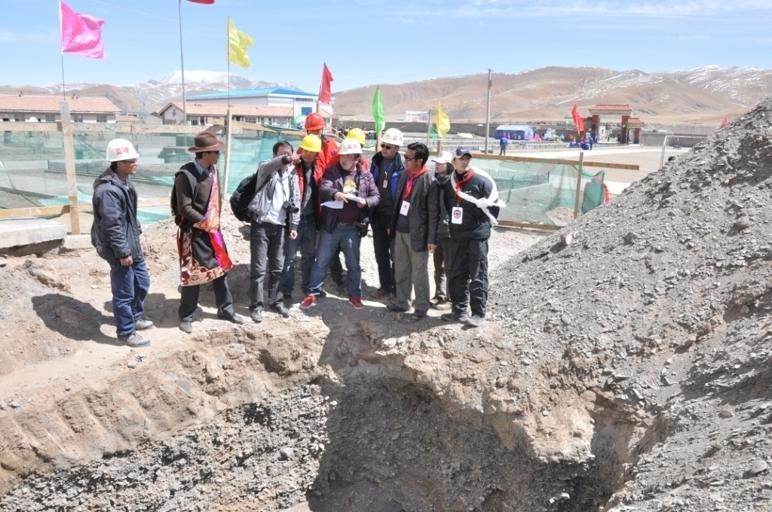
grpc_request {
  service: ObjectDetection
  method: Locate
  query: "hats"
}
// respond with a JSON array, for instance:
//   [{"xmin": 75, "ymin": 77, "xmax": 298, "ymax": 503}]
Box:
[
  {"xmin": 432, "ymin": 143, "xmax": 473, "ymax": 164},
  {"xmin": 188, "ymin": 132, "xmax": 225, "ymax": 152}
]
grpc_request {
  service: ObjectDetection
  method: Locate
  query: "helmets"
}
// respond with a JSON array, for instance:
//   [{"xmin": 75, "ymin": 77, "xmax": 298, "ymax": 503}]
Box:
[
  {"xmin": 106, "ymin": 138, "xmax": 140, "ymax": 162},
  {"xmin": 379, "ymin": 128, "xmax": 404, "ymax": 147},
  {"xmin": 299, "ymin": 113, "xmax": 365, "ymax": 155}
]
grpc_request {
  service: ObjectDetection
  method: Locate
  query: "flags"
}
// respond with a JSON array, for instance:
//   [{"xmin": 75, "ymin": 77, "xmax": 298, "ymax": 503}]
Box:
[
  {"xmin": 319, "ymin": 60, "xmax": 335, "ymax": 104},
  {"xmin": 228, "ymin": 17, "xmax": 255, "ymax": 71},
  {"xmin": 62, "ymin": 1, "xmax": 106, "ymax": 62},
  {"xmin": 436, "ymin": 102, "xmax": 450, "ymax": 138},
  {"xmin": 372, "ymin": 84, "xmax": 384, "ymax": 137},
  {"xmin": 188, "ymin": 0, "xmax": 216, "ymax": 5}
]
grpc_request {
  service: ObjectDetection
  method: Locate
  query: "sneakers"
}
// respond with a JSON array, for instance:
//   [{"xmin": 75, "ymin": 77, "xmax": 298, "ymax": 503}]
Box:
[
  {"xmin": 348, "ymin": 297, "xmax": 365, "ymax": 309},
  {"xmin": 299, "ymin": 294, "xmax": 317, "ymax": 309},
  {"xmin": 368, "ymin": 288, "xmax": 486, "ymax": 327}
]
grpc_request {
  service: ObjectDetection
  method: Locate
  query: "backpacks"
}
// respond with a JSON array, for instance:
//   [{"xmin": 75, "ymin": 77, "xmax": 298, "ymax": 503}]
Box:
[{"xmin": 230, "ymin": 167, "xmax": 277, "ymax": 223}]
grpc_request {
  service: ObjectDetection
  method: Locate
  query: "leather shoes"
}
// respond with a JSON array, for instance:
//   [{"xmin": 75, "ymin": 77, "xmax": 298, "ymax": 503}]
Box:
[
  {"xmin": 135, "ymin": 319, "xmax": 154, "ymax": 330},
  {"xmin": 217, "ymin": 313, "xmax": 245, "ymax": 323},
  {"xmin": 178, "ymin": 312, "xmax": 192, "ymax": 333},
  {"xmin": 250, "ymin": 301, "xmax": 289, "ymax": 322},
  {"xmin": 117, "ymin": 333, "xmax": 151, "ymax": 346}
]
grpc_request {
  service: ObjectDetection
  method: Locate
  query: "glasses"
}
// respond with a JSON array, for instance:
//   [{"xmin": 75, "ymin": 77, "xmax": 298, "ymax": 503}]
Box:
[
  {"xmin": 404, "ymin": 155, "xmax": 416, "ymax": 160},
  {"xmin": 381, "ymin": 144, "xmax": 392, "ymax": 149}
]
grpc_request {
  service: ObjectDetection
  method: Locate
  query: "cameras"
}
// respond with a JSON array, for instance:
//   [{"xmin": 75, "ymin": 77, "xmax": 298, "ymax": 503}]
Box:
[{"xmin": 283, "ymin": 201, "xmax": 299, "ymax": 213}]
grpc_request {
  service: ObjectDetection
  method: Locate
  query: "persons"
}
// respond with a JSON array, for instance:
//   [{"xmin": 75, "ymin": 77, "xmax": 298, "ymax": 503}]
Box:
[
  {"xmin": 441, "ymin": 146, "xmax": 500, "ymax": 328},
  {"xmin": 283, "ymin": 132, "xmax": 326, "ymax": 300},
  {"xmin": 578, "ymin": 133, "xmax": 600, "ymax": 149},
  {"xmin": 93, "ymin": 138, "xmax": 153, "ymax": 351},
  {"xmin": 297, "ymin": 113, "xmax": 341, "ymax": 285},
  {"xmin": 300, "ymin": 138, "xmax": 379, "ymax": 311},
  {"xmin": 170, "ymin": 131, "xmax": 242, "ymax": 333},
  {"xmin": 499, "ymin": 135, "xmax": 508, "ymax": 156},
  {"xmin": 371, "ymin": 127, "xmax": 404, "ymax": 299},
  {"xmin": 346, "ymin": 128, "xmax": 369, "ymax": 170},
  {"xmin": 429, "ymin": 150, "xmax": 455, "ymax": 304},
  {"xmin": 386, "ymin": 143, "xmax": 441, "ymax": 319},
  {"xmin": 248, "ymin": 140, "xmax": 301, "ymax": 322}
]
